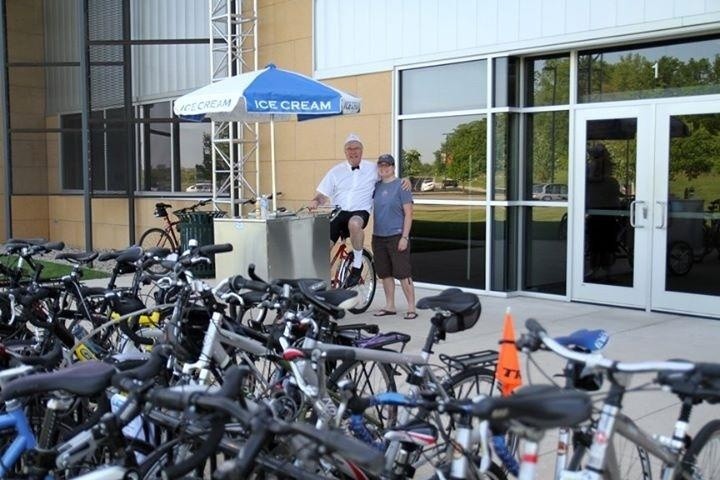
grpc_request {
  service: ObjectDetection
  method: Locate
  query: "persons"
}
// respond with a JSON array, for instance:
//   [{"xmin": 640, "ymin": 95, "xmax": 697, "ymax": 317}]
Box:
[
  {"xmin": 370, "ymin": 154, "xmax": 419, "ymax": 320},
  {"xmin": 585, "ymin": 140, "xmax": 636, "ymax": 285},
  {"xmin": 302, "ymin": 131, "xmax": 413, "ymax": 288}
]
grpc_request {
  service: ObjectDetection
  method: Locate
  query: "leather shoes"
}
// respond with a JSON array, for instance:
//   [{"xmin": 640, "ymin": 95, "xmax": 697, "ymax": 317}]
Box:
[{"xmin": 346, "ymin": 261, "xmax": 364, "ymax": 286}]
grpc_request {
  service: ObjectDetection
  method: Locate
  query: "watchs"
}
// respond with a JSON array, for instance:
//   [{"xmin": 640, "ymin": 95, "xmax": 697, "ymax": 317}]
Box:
[{"xmin": 401, "ymin": 235, "xmax": 410, "ymax": 241}]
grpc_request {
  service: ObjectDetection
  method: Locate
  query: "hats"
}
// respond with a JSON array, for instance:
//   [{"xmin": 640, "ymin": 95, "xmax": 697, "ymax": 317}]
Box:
[
  {"xmin": 377, "ymin": 153, "xmax": 395, "ymax": 166},
  {"xmin": 343, "ymin": 134, "xmax": 363, "ymax": 145}
]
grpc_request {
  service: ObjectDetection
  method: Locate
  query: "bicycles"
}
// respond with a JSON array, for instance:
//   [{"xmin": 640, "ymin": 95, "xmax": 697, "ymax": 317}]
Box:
[
  {"xmin": 243, "ymin": 195, "xmax": 287, "ymax": 214},
  {"xmin": 514, "ymin": 317, "xmax": 717, "ymax": 479},
  {"xmin": 1, "ymin": 237, "xmax": 648, "ymax": 480},
  {"xmin": 294, "ymin": 204, "xmax": 378, "ymax": 315},
  {"xmin": 135, "ymin": 199, "xmax": 212, "ymax": 276}
]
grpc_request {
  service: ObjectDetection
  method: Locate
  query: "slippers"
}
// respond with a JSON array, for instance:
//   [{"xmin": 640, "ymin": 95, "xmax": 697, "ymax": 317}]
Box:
[
  {"xmin": 404, "ymin": 311, "xmax": 418, "ymax": 320},
  {"xmin": 373, "ymin": 309, "xmax": 396, "ymax": 316}
]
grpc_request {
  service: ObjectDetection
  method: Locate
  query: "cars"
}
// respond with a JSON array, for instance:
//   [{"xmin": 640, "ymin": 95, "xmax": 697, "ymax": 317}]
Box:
[{"xmin": 532, "ymin": 184, "xmax": 569, "ymax": 201}]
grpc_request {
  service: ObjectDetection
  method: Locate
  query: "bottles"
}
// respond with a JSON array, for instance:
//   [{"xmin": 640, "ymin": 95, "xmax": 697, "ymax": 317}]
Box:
[
  {"xmin": 255, "ymin": 197, "xmax": 261, "ymax": 220},
  {"xmin": 73, "ymin": 324, "xmax": 103, "ymax": 355},
  {"xmin": 261, "ymin": 194, "xmax": 269, "ymax": 219}
]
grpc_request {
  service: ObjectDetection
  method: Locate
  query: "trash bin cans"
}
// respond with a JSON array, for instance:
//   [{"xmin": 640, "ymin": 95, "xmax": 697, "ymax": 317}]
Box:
[{"xmin": 176, "ymin": 211, "xmax": 231, "ymax": 278}]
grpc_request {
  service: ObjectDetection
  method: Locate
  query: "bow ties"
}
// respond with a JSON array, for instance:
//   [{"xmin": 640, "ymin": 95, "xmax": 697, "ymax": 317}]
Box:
[{"xmin": 352, "ymin": 166, "xmax": 359, "ymax": 170}]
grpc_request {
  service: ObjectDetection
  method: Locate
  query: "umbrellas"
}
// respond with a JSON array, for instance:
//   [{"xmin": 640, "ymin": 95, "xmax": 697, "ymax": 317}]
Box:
[
  {"xmin": 172, "ymin": 61, "xmax": 363, "ymax": 212},
  {"xmin": 582, "ymin": 111, "xmax": 692, "ymax": 194}
]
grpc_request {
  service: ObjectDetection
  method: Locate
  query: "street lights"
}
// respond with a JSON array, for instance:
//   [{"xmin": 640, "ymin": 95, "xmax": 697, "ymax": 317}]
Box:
[
  {"xmin": 443, "ymin": 132, "xmax": 453, "ymax": 188},
  {"xmin": 543, "ymin": 66, "xmax": 557, "ymax": 183}
]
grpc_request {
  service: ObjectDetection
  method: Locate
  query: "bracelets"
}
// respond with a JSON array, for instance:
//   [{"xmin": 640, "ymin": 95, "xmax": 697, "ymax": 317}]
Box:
[{"xmin": 312, "ymin": 198, "xmax": 320, "ymax": 205}]
